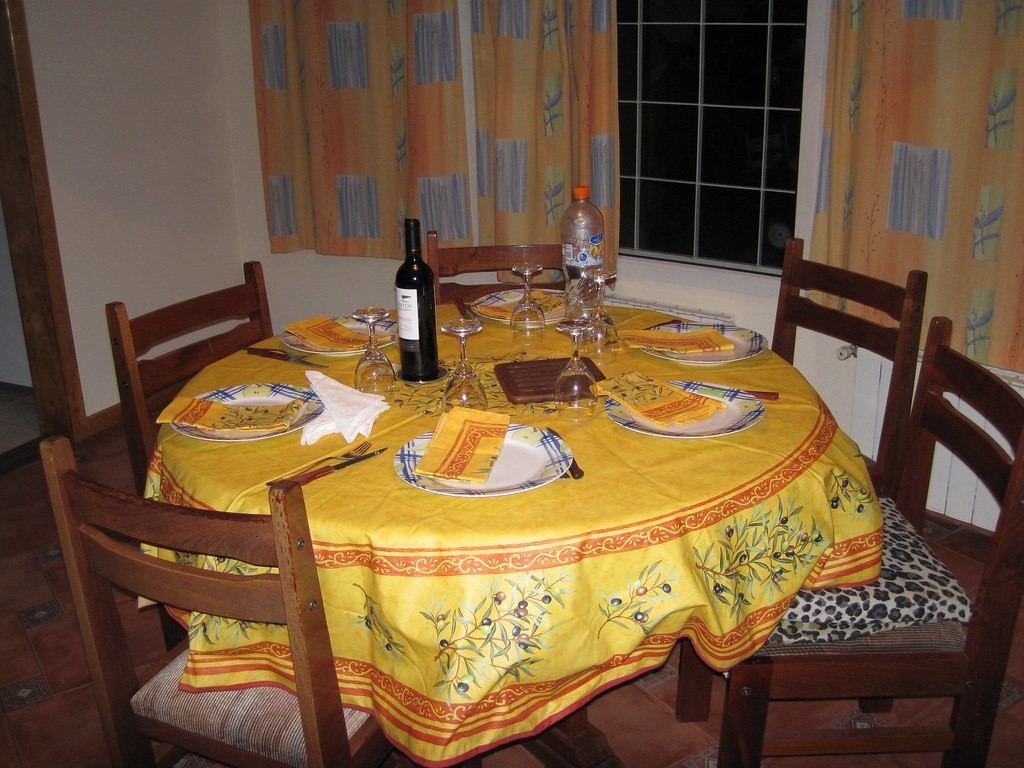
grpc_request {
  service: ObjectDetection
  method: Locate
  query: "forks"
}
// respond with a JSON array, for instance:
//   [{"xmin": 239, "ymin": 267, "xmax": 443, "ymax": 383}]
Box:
[{"xmin": 264, "ymin": 440, "xmax": 371, "ymax": 485}]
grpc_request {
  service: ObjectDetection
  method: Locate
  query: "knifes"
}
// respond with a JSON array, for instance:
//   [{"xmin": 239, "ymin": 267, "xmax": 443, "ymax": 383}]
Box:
[
  {"xmin": 453, "ymin": 295, "xmax": 470, "ymax": 320},
  {"xmin": 546, "ymin": 427, "xmax": 585, "ymax": 480},
  {"xmin": 247, "ymin": 347, "xmax": 329, "ymax": 369},
  {"xmin": 293, "ymin": 447, "xmax": 389, "ymax": 487}
]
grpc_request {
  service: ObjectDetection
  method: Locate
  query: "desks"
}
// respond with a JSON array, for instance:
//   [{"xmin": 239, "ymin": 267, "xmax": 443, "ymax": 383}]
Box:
[{"xmin": 138, "ymin": 302, "xmax": 882, "ymax": 768}]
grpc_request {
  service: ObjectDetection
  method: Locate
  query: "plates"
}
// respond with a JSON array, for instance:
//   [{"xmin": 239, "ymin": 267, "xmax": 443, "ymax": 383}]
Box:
[
  {"xmin": 394, "ymin": 421, "xmax": 574, "ymax": 498},
  {"xmin": 470, "ymin": 289, "xmax": 573, "ymax": 329},
  {"xmin": 278, "ymin": 319, "xmax": 399, "ymax": 357},
  {"xmin": 169, "ymin": 383, "xmax": 325, "ymax": 443},
  {"xmin": 604, "ymin": 381, "xmax": 764, "ymax": 439},
  {"xmin": 636, "ymin": 321, "xmax": 768, "ymax": 366}
]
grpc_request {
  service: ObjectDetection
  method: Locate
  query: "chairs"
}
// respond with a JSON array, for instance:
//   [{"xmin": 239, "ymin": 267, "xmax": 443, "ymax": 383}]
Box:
[
  {"xmin": 674, "ymin": 315, "xmax": 1024, "ymax": 768},
  {"xmin": 102, "ymin": 257, "xmax": 275, "ymax": 658},
  {"xmin": 425, "ymin": 230, "xmax": 599, "ymax": 303},
  {"xmin": 771, "ymin": 234, "xmax": 931, "ymax": 498},
  {"xmin": 39, "ymin": 432, "xmax": 389, "ymax": 768}
]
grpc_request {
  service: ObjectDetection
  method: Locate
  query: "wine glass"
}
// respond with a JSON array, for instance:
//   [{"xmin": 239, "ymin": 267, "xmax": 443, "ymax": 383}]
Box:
[
  {"xmin": 573, "ymin": 264, "xmax": 619, "ymax": 367},
  {"xmin": 511, "ymin": 259, "xmax": 546, "ymax": 345},
  {"xmin": 351, "ymin": 307, "xmax": 397, "ymax": 402},
  {"xmin": 553, "ymin": 317, "xmax": 599, "ymax": 421},
  {"xmin": 439, "ymin": 317, "xmax": 489, "ymax": 416}
]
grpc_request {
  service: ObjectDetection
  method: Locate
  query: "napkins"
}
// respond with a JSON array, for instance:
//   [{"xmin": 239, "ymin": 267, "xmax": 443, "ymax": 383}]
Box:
[
  {"xmin": 592, "ymin": 371, "xmax": 728, "ymax": 427},
  {"xmin": 618, "ymin": 325, "xmax": 735, "ymax": 351},
  {"xmin": 283, "ymin": 315, "xmax": 393, "ymax": 351},
  {"xmin": 300, "ymin": 369, "xmax": 390, "ymax": 445},
  {"xmin": 155, "ymin": 393, "xmax": 303, "ymax": 429},
  {"xmin": 474, "ymin": 289, "xmax": 565, "ymax": 318},
  {"xmin": 413, "ymin": 402, "xmax": 509, "ymax": 481}
]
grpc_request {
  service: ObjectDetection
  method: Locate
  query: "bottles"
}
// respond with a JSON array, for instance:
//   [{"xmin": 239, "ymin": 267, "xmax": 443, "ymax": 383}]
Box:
[
  {"xmin": 392, "ymin": 218, "xmax": 440, "ymax": 383},
  {"xmin": 560, "ymin": 186, "xmax": 607, "ymax": 322}
]
